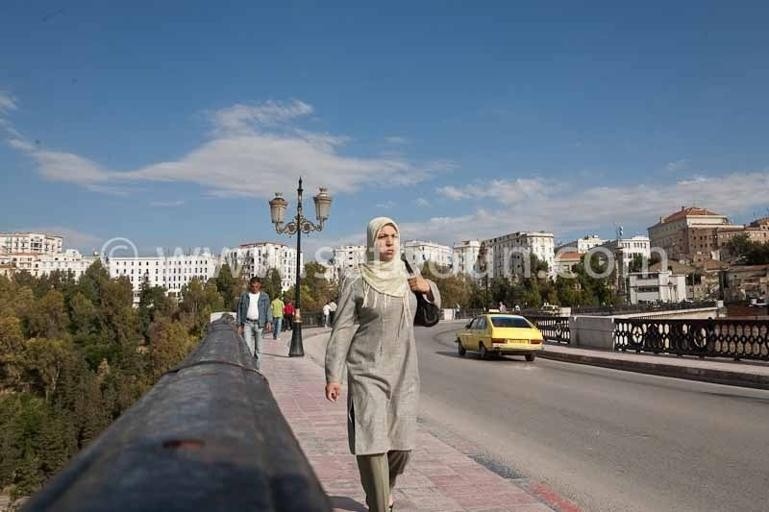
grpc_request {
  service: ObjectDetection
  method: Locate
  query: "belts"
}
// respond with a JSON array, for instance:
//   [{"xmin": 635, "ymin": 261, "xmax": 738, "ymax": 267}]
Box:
[{"xmin": 246, "ymin": 318, "xmax": 258, "ymax": 322}]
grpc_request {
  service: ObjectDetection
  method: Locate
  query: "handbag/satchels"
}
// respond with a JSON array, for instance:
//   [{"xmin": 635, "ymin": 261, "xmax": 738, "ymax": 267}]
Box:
[{"xmin": 403, "ymin": 258, "xmax": 439, "ymax": 327}]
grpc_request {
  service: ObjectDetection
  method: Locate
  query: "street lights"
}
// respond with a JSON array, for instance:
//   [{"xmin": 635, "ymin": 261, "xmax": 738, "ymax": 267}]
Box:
[{"xmin": 268, "ymin": 176, "xmax": 331, "ymax": 357}]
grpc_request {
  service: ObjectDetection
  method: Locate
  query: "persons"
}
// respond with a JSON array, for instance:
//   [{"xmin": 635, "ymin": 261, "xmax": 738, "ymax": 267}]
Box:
[
  {"xmin": 237, "ymin": 277, "xmax": 271, "ymax": 373},
  {"xmin": 269, "ymin": 293, "xmax": 294, "ymax": 340},
  {"xmin": 325, "ymin": 217, "xmax": 441, "ymax": 512},
  {"xmin": 498, "ymin": 301, "xmax": 506, "ymax": 312},
  {"xmin": 511, "ymin": 303, "xmax": 521, "ymax": 314},
  {"xmin": 323, "ymin": 299, "xmax": 337, "ymax": 328},
  {"xmin": 456, "ymin": 302, "xmax": 461, "ymax": 320}
]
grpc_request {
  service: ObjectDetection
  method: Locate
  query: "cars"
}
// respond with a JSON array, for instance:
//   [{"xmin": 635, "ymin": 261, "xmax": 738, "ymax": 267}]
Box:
[{"xmin": 456, "ymin": 309, "xmax": 544, "ymax": 361}]
